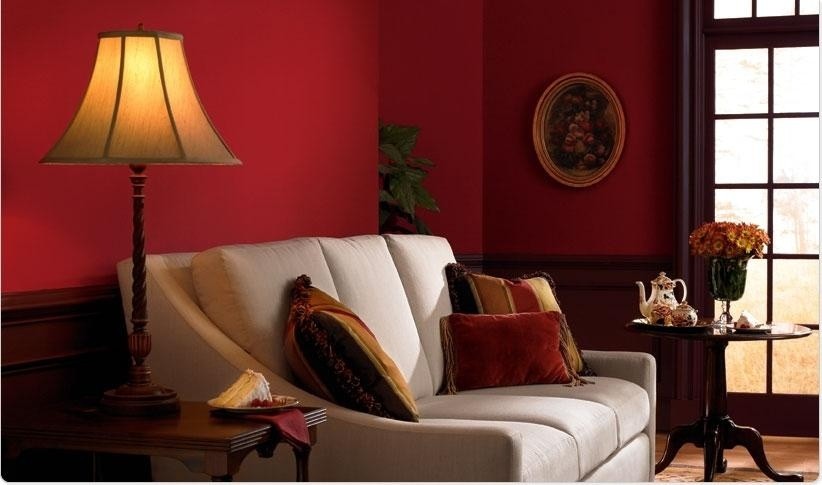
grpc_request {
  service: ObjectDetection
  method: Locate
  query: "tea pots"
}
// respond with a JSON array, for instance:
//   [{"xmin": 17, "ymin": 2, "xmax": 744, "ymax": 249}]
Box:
[{"xmin": 635, "ymin": 272, "xmax": 698, "ymax": 326}]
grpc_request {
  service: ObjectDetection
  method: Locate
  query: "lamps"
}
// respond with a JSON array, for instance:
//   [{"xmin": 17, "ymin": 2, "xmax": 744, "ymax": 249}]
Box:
[{"xmin": 38, "ymin": 21, "xmax": 243, "ymax": 419}]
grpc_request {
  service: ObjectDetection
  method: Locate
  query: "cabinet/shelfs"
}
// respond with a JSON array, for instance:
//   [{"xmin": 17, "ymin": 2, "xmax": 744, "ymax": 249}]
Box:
[{"xmin": 1, "ymin": 401, "xmax": 328, "ymax": 482}]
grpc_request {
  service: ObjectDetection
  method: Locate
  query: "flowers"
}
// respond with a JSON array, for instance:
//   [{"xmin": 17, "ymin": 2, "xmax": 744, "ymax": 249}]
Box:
[{"xmin": 688, "ymin": 221, "xmax": 772, "ymax": 260}]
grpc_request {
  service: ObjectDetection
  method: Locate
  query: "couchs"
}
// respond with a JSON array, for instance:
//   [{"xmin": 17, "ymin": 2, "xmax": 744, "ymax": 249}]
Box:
[{"xmin": 116, "ymin": 231, "xmax": 659, "ymax": 482}]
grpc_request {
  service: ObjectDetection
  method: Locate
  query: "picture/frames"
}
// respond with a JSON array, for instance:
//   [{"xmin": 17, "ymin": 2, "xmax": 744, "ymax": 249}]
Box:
[{"xmin": 532, "ymin": 71, "xmax": 626, "ymax": 188}]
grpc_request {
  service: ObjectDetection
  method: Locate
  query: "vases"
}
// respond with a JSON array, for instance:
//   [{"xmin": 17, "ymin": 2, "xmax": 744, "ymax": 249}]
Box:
[{"xmin": 704, "ymin": 256, "xmax": 750, "ymax": 327}]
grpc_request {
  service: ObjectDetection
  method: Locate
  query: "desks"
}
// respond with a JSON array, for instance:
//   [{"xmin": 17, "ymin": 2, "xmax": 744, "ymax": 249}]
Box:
[{"xmin": 626, "ymin": 318, "xmax": 810, "ymax": 482}]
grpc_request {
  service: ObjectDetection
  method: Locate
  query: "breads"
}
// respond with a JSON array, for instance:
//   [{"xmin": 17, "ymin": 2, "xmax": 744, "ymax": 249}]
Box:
[
  {"xmin": 737, "ymin": 312, "xmax": 761, "ymax": 329},
  {"xmin": 208, "ymin": 369, "xmax": 273, "ymax": 408}
]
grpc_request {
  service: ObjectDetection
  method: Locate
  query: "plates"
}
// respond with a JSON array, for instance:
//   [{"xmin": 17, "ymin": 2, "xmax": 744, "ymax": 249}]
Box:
[
  {"xmin": 206, "ymin": 394, "xmax": 298, "ymax": 412},
  {"xmin": 727, "ymin": 324, "xmax": 772, "ymax": 331}
]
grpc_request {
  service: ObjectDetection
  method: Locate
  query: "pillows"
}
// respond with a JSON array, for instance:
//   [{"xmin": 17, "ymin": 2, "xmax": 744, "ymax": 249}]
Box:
[
  {"xmin": 317, "ymin": 235, "xmax": 433, "ymax": 399},
  {"xmin": 438, "ymin": 311, "xmax": 594, "ymax": 400},
  {"xmin": 384, "ymin": 234, "xmax": 457, "ymax": 396},
  {"xmin": 283, "ymin": 273, "xmax": 419, "ymax": 424},
  {"xmin": 190, "ymin": 237, "xmax": 339, "ymax": 383},
  {"xmin": 445, "ymin": 263, "xmax": 598, "ymax": 377}
]
